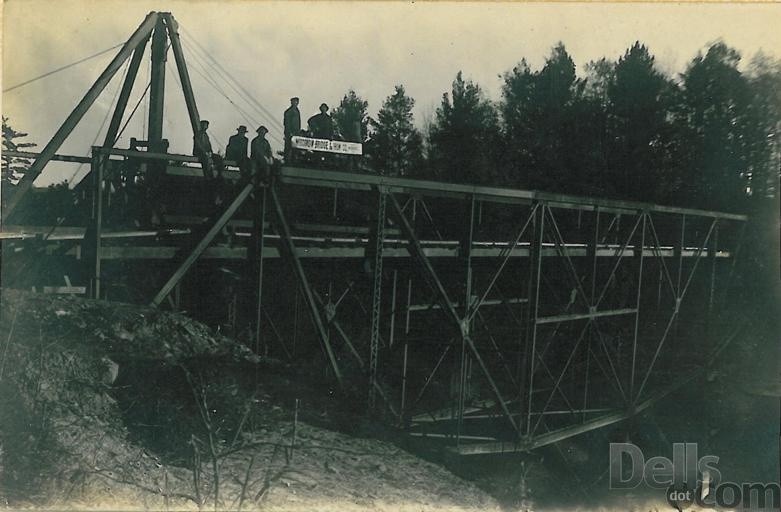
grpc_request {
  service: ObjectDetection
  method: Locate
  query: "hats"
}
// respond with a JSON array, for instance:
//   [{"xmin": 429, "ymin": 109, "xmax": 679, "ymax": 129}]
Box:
[
  {"xmin": 319, "ymin": 103, "xmax": 328, "ymax": 111},
  {"xmin": 255, "ymin": 125, "xmax": 268, "ymax": 133},
  {"xmin": 236, "ymin": 125, "xmax": 248, "ymax": 132}
]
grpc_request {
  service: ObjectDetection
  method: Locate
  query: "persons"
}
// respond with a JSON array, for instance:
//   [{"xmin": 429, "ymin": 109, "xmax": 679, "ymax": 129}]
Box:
[
  {"xmin": 226, "ymin": 126, "xmax": 252, "ymax": 187},
  {"xmin": 284, "ymin": 96, "xmax": 302, "ymax": 163},
  {"xmin": 309, "ymin": 103, "xmax": 335, "ymax": 143},
  {"xmin": 192, "ymin": 120, "xmax": 223, "ymax": 184},
  {"xmin": 253, "ymin": 126, "xmax": 277, "ymax": 191}
]
